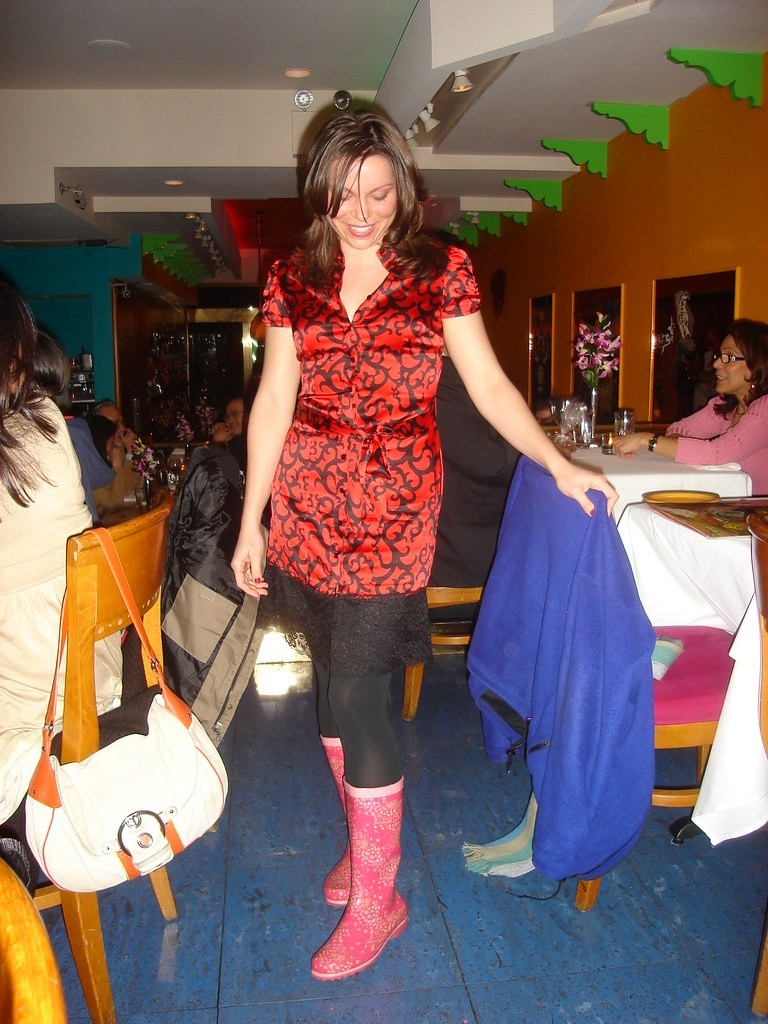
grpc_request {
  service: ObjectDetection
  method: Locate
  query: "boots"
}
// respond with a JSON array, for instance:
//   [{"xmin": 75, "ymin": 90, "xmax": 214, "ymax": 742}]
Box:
[
  {"xmin": 320, "ymin": 730, "xmax": 355, "ymax": 905},
  {"xmin": 311, "ymin": 775, "xmax": 408, "ymax": 981}
]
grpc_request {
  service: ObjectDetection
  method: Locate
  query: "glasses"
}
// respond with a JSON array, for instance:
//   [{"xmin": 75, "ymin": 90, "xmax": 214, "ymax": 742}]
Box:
[
  {"xmin": 223, "ymin": 410, "xmax": 250, "ymax": 423},
  {"xmin": 713, "ymin": 351, "xmax": 746, "ymax": 365}
]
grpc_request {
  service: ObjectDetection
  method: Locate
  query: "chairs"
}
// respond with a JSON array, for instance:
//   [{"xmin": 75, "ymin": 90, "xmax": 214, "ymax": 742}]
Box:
[
  {"xmin": 469, "ymin": 454, "xmax": 737, "ymax": 914},
  {"xmin": 744, "ymin": 510, "xmax": 768, "ymax": 1020},
  {"xmin": 401, "ymin": 585, "xmax": 484, "ymax": 722},
  {"xmin": 0, "ymin": 486, "xmax": 175, "ymax": 1024}
]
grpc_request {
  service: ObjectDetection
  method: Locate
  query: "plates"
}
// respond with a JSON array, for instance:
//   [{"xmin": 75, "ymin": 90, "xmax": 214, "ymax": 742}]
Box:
[{"xmin": 643, "ymin": 491, "xmax": 720, "ymax": 505}]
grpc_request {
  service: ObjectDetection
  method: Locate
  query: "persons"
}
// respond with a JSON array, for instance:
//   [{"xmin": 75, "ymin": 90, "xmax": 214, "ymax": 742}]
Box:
[
  {"xmin": 229, "ymin": 112, "xmax": 618, "ymax": 979},
  {"xmin": 0, "ymin": 268, "xmax": 122, "ymax": 829},
  {"xmin": 423, "ymin": 341, "xmax": 524, "ymax": 624},
  {"xmin": 29, "ymin": 328, "xmax": 274, "ymax": 532},
  {"xmin": 609, "ymin": 318, "xmax": 768, "ymax": 498}
]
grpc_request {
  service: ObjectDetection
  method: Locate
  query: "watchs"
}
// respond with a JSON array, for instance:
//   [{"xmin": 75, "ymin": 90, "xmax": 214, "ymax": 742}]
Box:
[{"xmin": 648, "ymin": 432, "xmax": 661, "ymax": 451}]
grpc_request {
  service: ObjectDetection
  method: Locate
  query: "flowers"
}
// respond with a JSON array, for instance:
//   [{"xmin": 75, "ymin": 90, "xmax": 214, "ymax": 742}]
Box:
[
  {"xmin": 571, "ymin": 310, "xmax": 622, "ymax": 388},
  {"xmin": 130, "ymin": 437, "xmax": 160, "ymax": 481},
  {"xmin": 194, "ymin": 401, "xmax": 214, "ymax": 427},
  {"xmin": 174, "ymin": 410, "xmax": 195, "ymax": 441}
]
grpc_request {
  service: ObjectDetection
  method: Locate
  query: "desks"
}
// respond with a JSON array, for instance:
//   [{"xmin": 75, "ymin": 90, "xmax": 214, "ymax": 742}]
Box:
[
  {"xmin": 96, "ymin": 499, "xmax": 150, "ymax": 528},
  {"xmin": 568, "ymin": 441, "xmax": 768, "ymax": 844}
]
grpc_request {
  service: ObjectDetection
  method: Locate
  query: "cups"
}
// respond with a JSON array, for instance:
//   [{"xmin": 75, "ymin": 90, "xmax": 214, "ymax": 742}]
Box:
[
  {"xmin": 81, "ymin": 354, "xmax": 92, "ymax": 370},
  {"xmin": 550, "ymin": 399, "xmax": 572, "ymax": 426},
  {"xmin": 614, "ymin": 410, "xmax": 635, "ymax": 435},
  {"xmin": 78, "ymin": 374, "xmax": 85, "ymax": 381},
  {"xmin": 602, "ymin": 432, "xmax": 613, "ymax": 454},
  {"xmin": 168, "ymin": 457, "xmax": 182, "ymax": 474},
  {"xmin": 135, "ymin": 487, "xmax": 150, "ymax": 514}
]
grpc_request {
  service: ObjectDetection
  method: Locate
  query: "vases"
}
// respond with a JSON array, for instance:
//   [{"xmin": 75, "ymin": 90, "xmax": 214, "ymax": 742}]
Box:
[
  {"xmin": 585, "ymin": 385, "xmax": 600, "ymax": 442},
  {"xmin": 185, "ymin": 442, "xmax": 191, "ymax": 461},
  {"xmin": 207, "ymin": 426, "xmax": 212, "ymax": 440},
  {"xmin": 144, "ymin": 479, "xmax": 151, "ymax": 505}
]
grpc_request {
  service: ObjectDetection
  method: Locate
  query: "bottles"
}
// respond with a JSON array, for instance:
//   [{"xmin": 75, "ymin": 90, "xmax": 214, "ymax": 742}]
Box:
[{"xmin": 578, "ymin": 410, "xmax": 591, "ymax": 443}]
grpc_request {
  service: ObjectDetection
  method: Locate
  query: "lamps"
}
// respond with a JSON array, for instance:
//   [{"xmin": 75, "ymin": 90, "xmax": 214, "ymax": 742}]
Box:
[
  {"xmin": 185, "ymin": 212, "xmax": 227, "ymax": 271},
  {"xmin": 419, "ymin": 103, "xmax": 441, "ymax": 133},
  {"xmin": 290, "ymin": 88, "xmax": 353, "ymax": 158},
  {"xmin": 451, "ymin": 69, "xmax": 474, "ymax": 92},
  {"xmin": 404, "ymin": 125, "xmax": 419, "ymax": 150}
]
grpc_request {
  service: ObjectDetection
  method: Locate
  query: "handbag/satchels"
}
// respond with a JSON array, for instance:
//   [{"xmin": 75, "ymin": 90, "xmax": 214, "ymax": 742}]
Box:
[{"xmin": 24, "ymin": 527, "xmax": 229, "ymax": 893}]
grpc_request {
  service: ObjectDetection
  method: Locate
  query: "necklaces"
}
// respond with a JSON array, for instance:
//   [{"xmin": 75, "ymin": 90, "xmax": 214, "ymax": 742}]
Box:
[{"xmin": 736, "ymin": 405, "xmax": 746, "ymax": 415}]
